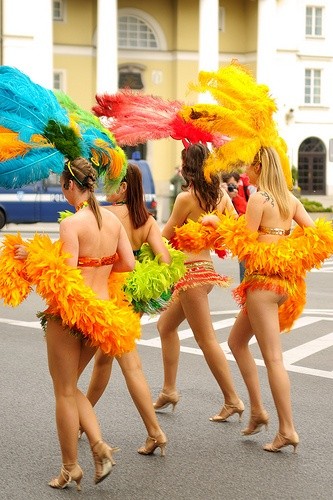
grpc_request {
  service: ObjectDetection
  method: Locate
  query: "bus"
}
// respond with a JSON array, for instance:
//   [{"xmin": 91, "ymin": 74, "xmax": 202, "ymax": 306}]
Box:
[{"xmin": 0, "ymin": 151, "xmax": 157, "ymax": 229}]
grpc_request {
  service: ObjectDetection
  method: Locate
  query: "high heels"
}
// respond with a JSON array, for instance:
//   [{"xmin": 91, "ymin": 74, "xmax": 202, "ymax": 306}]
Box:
[
  {"xmin": 263, "ymin": 431, "xmax": 300, "ymax": 452},
  {"xmin": 209, "ymin": 399, "xmax": 245, "ymax": 423},
  {"xmin": 137, "ymin": 432, "xmax": 166, "ymax": 456},
  {"xmin": 48, "ymin": 462, "xmax": 84, "ymax": 491},
  {"xmin": 153, "ymin": 391, "xmax": 179, "ymax": 412},
  {"xmin": 241, "ymin": 410, "xmax": 270, "ymax": 435},
  {"xmin": 90, "ymin": 438, "xmax": 121, "ymax": 485}
]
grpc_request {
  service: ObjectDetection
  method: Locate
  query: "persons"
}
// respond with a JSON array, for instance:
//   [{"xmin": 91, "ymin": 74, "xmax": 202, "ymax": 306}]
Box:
[
  {"xmin": 153, "ymin": 144, "xmax": 245, "ymax": 424},
  {"xmin": 80, "ymin": 163, "xmax": 171, "ymax": 456},
  {"xmin": 227, "ymin": 146, "xmax": 316, "ymax": 454},
  {"xmin": 14, "ymin": 157, "xmax": 135, "ymax": 490},
  {"xmin": 170, "ymin": 166, "xmax": 185, "ymax": 205},
  {"xmin": 220, "ymin": 171, "xmax": 255, "ymax": 285}
]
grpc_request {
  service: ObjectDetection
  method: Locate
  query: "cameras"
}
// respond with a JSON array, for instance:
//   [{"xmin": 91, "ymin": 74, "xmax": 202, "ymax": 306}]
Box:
[{"xmin": 227, "ymin": 183, "xmax": 237, "ymax": 192}]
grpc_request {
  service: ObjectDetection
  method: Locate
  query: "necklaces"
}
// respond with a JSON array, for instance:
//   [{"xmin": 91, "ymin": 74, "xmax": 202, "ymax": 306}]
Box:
[{"xmin": 75, "ymin": 199, "xmax": 89, "ymax": 212}]
